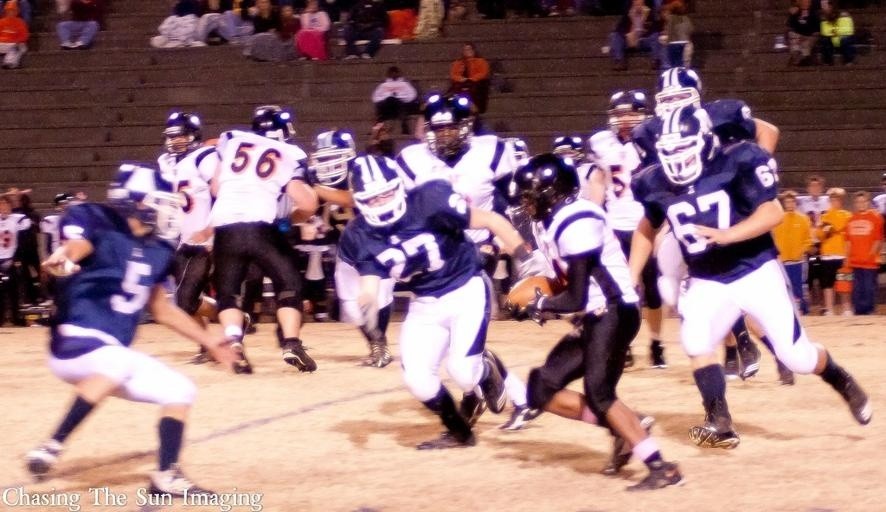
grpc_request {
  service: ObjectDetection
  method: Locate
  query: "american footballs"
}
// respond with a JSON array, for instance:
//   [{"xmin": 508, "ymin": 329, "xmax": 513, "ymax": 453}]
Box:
[{"xmin": 504, "ymin": 276, "xmax": 565, "ymax": 309}]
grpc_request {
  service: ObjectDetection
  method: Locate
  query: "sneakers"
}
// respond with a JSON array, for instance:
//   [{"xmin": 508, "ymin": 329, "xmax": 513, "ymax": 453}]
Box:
[
  {"xmin": 25, "ymin": 309, "xmax": 874, "ymax": 500},
  {"xmin": 60, "ymin": 40, "xmax": 73, "ymax": 49},
  {"xmin": 71, "ymin": 40, "xmax": 86, "ymax": 50}
]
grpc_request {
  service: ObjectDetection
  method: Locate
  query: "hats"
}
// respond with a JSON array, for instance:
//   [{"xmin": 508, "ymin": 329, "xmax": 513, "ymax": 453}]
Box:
[{"xmin": 4, "ymin": 2, "xmax": 17, "ymax": 11}]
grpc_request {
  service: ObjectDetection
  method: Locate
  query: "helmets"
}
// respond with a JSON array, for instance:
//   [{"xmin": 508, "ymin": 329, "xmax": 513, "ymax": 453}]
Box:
[
  {"xmin": 553, "ymin": 136, "xmax": 589, "ymax": 167},
  {"xmin": 106, "ymin": 160, "xmax": 187, "ymax": 240},
  {"xmin": 252, "ymin": 105, "xmax": 296, "ymax": 143},
  {"xmin": 507, "ymin": 151, "xmax": 579, "ymax": 226},
  {"xmin": 348, "ymin": 153, "xmax": 407, "ymax": 226},
  {"xmin": 161, "ymin": 111, "xmax": 204, "ymax": 155},
  {"xmin": 422, "ymin": 90, "xmax": 478, "ymax": 158},
  {"xmin": 608, "ymin": 90, "xmax": 654, "ymax": 134},
  {"xmin": 502, "ymin": 138, "xmax": 530, "ymax": 166},
  {"xmin": 310, "ymin": 130, "xmax": 357, "ymax": 185},
  {"xmin": 54, "ymin": 194, "xmax": 71, "ymax": 211},
  {"xmin": 654, "ymin": 67, "xmax": 702, "ymax": 118},
  {"xmin": 655, "ymin": 105, "xmax": 722, "ymax": 185}
]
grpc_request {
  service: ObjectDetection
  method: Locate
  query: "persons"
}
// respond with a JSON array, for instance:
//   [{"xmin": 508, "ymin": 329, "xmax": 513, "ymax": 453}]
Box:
[
  {"xmin": 24, "ymin": 162, "xmax": 244, "ymax": 501},
  {"xmin": 393, "ymin": 96, "xmax": 544, "ymax": 433},
  {"xmin": 334, "ymin": 156, "xmax": 544, "ymax": 449},
  {"xmin": 606, "ymin": 0, "xmax": 661, "ymax": 71},
  {"xmin": 2, "ymin": 190, "xmax": 40, "ymax": 326},
  {"xmin": 629, "ymin": 107, "xmax": 871, "ymax": 450},
  {"xmin": 777, "ymin": 0, "xmax": 817, "ymax": 67},
  {"xmin": 40, "ymin": 192, "xmax": 87, "ymax": 256},
  {"xmin": 627, "ymin": 67, "xmax": 796, "ymax": 387},
  {"xmin": 553, "ymin": 137, "xmax": 607, "ymax": 208},
  {"xmin": 0, "ymin": 0, "xmax": 30, "ymax": 68},
  {"xmin": 54, "ymin": 1, "xmax": 101, "ymax": 49},
  {"xmin": 149, "ymin": 1, "xmax": 445, "ymax": 63},
  {"xmin": 446, "ymin": 1, "xmax": 604, "ymax": 18},
  {"xmin": 3, "ymin": 186, "xmax": 41, "ymax": 306},
  {"xmin": 498, "ymin": 151, "xmax": 686, "ymax": 490},
  {"xmin": 870, "ymin": 173, "xmax": 886, "ymax": 225},
  {"xmin": 647, "ymin": 0, "xmax": 702, "ymax": 70},
  {"xmin": 797, "ymin": 173, "xmax": 830, "ymax": 301},
  {"xmin": 844, "ymin": 190, "xmax": 885, "ymax": 316},
  {"xmin": 301, "ymin": 251, "xmax": 332, "ymax": 319},
  {"xmin": 770, "ymin": 191, "xmax": 813, "ymax": 316},
  {"xmin": 450, "ymin": 42, "xmax": 490, "ymax": 115},
  {"xmin": 210, "ymin": 105, "xmax": 317, "ymax": 374},
  {"xmin": 308, "ymin": 129, "xmax": 395, "ymax": 368},
  {"xmin": 591, "ymin": 92, "xmax": 667, "ymax": 371},
  {"xmin": 816, "ymin": 0, "xmax": 864, "ymax": 70},
  {"xmin": 368, "ymin": 67, "xmax": 419, "ymax": 135},
  {"xmin": 157, "ymin": 110, "xmax": 256, "ymax": 366},
  {"xmin": 816, "ymin": 187, "xmax": 854, "ymax": 316}
]
